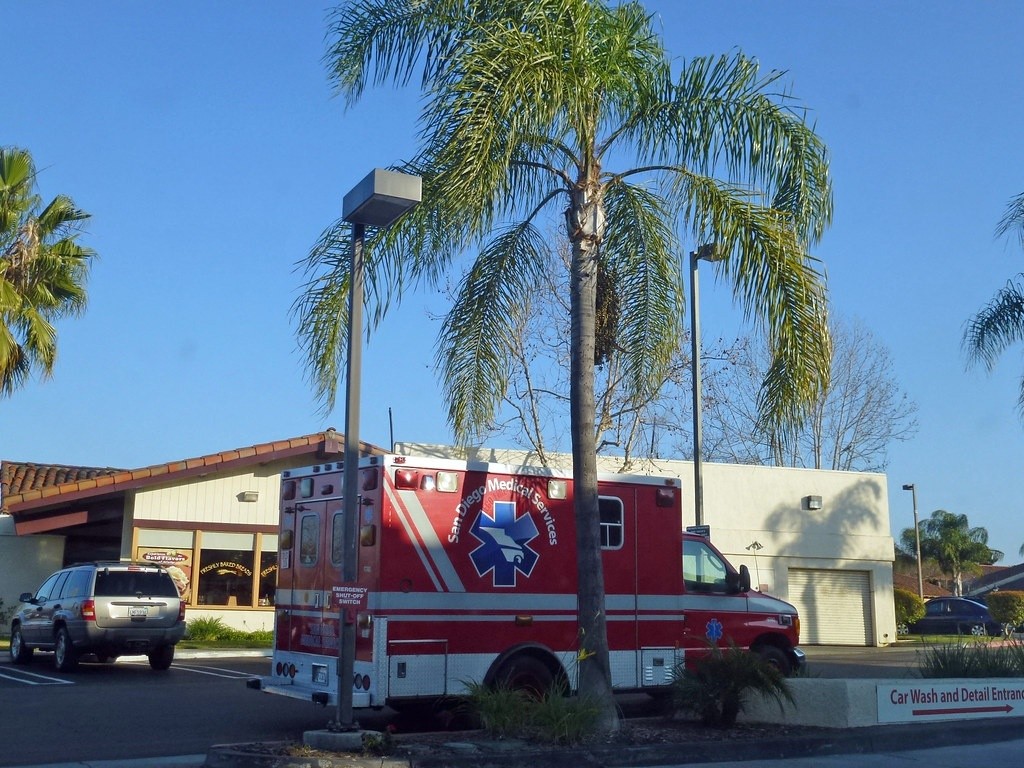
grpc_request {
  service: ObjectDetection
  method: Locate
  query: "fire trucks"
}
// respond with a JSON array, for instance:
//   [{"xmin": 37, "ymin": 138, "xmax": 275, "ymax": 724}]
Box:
[{"xmin": 260, "ymin": 461, "xmax": 804, "ymax": 713}]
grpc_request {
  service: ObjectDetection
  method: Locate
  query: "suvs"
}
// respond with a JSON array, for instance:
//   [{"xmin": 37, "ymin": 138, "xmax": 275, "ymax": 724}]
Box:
[{"xmin": 8, "ymin": 558, "xmax": 185, "ymax": 671}]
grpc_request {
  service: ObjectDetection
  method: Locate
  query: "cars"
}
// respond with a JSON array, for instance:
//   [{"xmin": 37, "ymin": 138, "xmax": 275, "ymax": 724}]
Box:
[{"xmin": 897, "ymin": 596, "xmax": 1004, "ymax": 638}]
